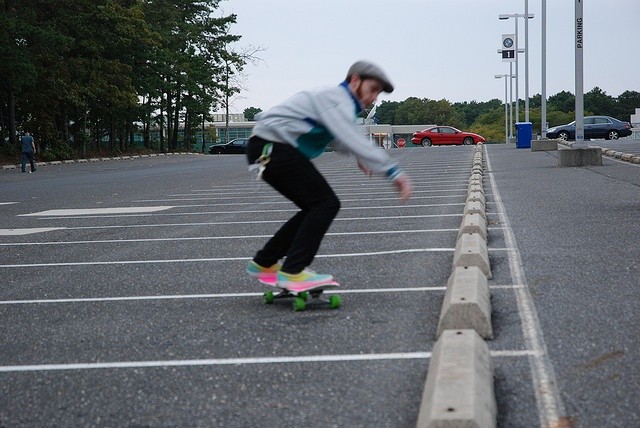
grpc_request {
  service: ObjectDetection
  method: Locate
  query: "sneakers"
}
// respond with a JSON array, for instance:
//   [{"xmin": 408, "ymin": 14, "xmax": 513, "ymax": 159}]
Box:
[
  {"xmin": 245, "ymin": 260, "xmax": 283, "ymax": 276},
  {"xmin": 276, "ymin": 268, "xmax": 333, "ymax": 291}
]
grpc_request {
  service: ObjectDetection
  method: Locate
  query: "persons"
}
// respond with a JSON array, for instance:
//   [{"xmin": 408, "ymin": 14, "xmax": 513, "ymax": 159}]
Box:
[
  {"xmin": 242, "ymin": 58, "xmax": 414, "ymax": 292},
  {"xmin": 18, "ymin": 130, "xmax": 38, "ymax": 173}
]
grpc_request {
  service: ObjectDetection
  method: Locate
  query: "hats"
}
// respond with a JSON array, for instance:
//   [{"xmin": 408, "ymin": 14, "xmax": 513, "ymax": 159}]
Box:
[{"xmin": 347, "ymin": 61, "xmax": 393, "ymax": 93}]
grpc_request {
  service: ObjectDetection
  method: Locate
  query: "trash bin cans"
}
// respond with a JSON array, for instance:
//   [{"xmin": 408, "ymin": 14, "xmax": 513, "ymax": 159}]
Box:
[{"xmin": 515, "ymin": 122, "xmax": 532, "ymax": 148}]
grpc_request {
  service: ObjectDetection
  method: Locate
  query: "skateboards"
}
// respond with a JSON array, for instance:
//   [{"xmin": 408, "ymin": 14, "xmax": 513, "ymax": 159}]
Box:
[{"xmin": 256, "ymin": 266, "xmax": 342, "ymax": 311}]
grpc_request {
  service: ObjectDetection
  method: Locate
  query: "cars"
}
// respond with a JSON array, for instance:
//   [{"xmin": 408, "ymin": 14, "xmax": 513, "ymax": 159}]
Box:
[
  {"xmin": 546, "ymin": 116, "xmax": 632, "ymax": 141},
  {"xmin": 411, "ymin": 126, "xmax": 485, "ymax": 147},
  {"xmin": 208, "ymin": 138, "xmax": 249, "ymax": 154}
]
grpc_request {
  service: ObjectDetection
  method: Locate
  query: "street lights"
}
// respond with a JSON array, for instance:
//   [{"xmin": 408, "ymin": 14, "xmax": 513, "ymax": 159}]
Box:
[
  {"xmin": 494, "ymin": 74, "xmax": 517, "ymax": 144},
  {"xmin": 497, "ymin": 49, "xmax": 524, "ymax": 122},
  {"xmin": 499, "ymin": 13, "xmax": 534, "ymax": 122}
]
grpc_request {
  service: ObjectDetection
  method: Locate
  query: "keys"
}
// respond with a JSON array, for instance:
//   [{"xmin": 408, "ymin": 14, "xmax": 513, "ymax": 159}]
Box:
[{"xmin": 246, "ymin": 161, "xmax": 267, "ymax": 182}]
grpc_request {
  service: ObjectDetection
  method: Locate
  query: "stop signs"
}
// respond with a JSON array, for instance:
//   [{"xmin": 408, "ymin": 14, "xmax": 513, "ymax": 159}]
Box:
[{"xmin": 397, "ymin": 138, "xmax": 406, "ymax": 148}]
